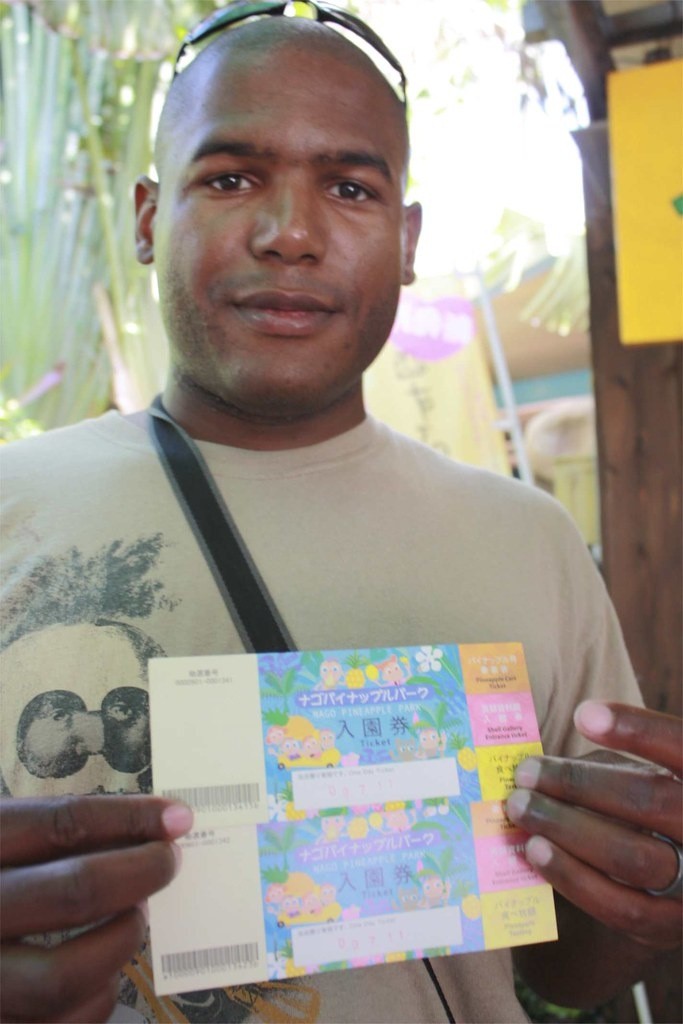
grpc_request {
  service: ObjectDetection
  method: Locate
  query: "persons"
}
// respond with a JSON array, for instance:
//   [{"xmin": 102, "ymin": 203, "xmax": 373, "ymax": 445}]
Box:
[{"xmin": 0, "ymin": 0, "xmax": 683, "ymax": 1024}]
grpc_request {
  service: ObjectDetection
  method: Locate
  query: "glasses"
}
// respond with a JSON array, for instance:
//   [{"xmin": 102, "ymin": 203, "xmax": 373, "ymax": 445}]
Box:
[{"xmin": 171, "ymin": 4, "xmax": 408, "ymax": 93}]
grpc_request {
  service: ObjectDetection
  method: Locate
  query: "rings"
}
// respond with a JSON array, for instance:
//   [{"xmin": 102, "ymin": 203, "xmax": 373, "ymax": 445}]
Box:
[{"xmin": 644, "ymin": 834, "xmax": 683, "ymax": 901}]
